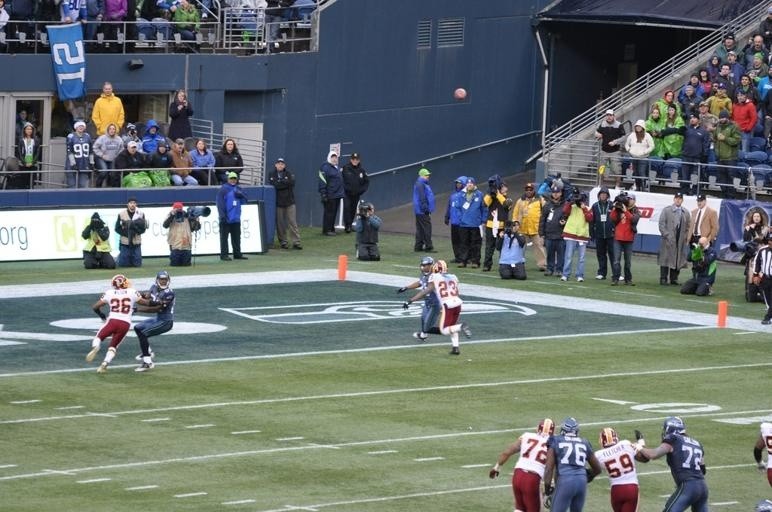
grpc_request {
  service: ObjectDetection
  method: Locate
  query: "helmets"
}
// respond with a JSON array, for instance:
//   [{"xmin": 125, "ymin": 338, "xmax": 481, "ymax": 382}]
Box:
[
  {"xmin": 662, "ymin": 417, "xmax": 686, "ymax": 438},
  {"xmin": 755, "ymin": 499, "xmax": 772, "ymax": 512},
  {"xmin": 537, "ymin": 417, "xmax": 578, "ymax": 436},
  {"xmin": 156, "ymin": 270, "xmax": 170, "ymax": 289},
  {"xmin": 420, "ymin": 256, "xmax": 448, "ymax": 275},
  {"xmin": 599, "ymin": 427, "xmax": 617, "ymax": 447},
  {"xmin": 112, "ymin": 274, "xmax": 129, "ymax": 289}
]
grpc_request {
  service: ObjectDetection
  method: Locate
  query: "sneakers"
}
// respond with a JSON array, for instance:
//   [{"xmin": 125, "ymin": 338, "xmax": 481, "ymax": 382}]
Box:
[
  {"xmin": 461, "ymin": 322, "xmax": 472, "ymax": 339},
  {"xmin": 86, "ymin": 346, "xmax": 99, "ymax": 361},
  {"xmin": 413, "ymin": 332, "xmax": 428, "ymax": 341},
  {"xmin": 544, "ymin": 270, "xmax": 584, "ymax": 282},
  {"xmin": 281, "ymin": 243, "xmax": 303, "ymax": 249},
  {"xmin": 596, "ymin": 275, "xmax": 635, "ymax": 285},
  {"xmin": 97, "ymin": 362, "xmax": 107, "ymax": 372},
  {"xmin": 324, "ymin": 226, "xmax": 354, "ymax": 236},
  {"xmin": 449, "ymin": 349, "xmax": 459, "ymax": 354},
  {"xmin": 415, "ymin": 247, "xmax": 437, "ymax": 252},
  {"xmin": 458, "ymin": 263, "xmax": 490, "ymax": 271},
  {"xmin": 134, "ymin": 350, "xmax": 154, "ymax": 372}
]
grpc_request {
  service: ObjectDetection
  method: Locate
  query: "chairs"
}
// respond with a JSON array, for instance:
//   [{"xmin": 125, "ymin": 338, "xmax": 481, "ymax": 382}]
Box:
[
  {"xmin": 618, "ymin": 40, "xmax": 772, "ymax": 197},
  {"xmin": 0, "ymin": 0, "xmax": 318, "ymax": 54},
  {"xmin": 80, "ymin": 121, "xmax": 203, "ymax": 186}
]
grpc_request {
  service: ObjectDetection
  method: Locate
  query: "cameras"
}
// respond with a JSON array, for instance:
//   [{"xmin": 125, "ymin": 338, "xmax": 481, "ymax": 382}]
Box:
[
  {"xmin": 91, "ymin": 219, "xmax": 99, "ymax": 225},
  {"xmin": 175, "ymin": 209, "xmax": 183, "ymax": 216}
]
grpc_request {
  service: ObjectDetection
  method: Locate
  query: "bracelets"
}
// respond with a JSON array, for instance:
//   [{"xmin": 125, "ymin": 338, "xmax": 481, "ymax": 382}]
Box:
[
  {"xmin": 638, "ymin": 439, "xmax": 645, "ymax": 446},
  {"xmin": 636, "ymin": 444, "xmax": 642, "ymax": 451},
  {"xmin": 493, "ymin": 462, "xmax": 502, "ymax": 472}
]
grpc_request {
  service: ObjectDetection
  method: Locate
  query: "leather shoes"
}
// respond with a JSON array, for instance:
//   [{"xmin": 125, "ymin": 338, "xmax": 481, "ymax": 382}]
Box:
[
  {"xmin": 660, "ymin": 281, "xmax": 678, "ymax": 285},
  {"xmin": 221, "ymin": 255, "xmax": 247, "ymax": 260}
]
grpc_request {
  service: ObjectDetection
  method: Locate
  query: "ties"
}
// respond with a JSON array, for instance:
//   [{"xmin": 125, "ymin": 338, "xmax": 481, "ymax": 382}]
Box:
[{"xmin": 694, "ymin": 210, "xmax": 700, "ymax": 234}]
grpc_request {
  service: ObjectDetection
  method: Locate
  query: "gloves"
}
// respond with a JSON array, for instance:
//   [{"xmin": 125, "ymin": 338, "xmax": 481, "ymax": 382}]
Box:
[
  {"xmin": 403, "ymin": 299, "xmax": 412, "ymax": 308},
  {"xmin": 398, "ymin": 287, "xmax": 407, "ymax": 292},
  {"xmin": 635, "ymin": 430, "xmax": 645, "ymax": 446},
  {"xmin": 758, "ymin": 461, "xmax": 766, "ymax": 473},
  {"xmin": 490, "ymin": 464, "xmax": 501, "ymax": 478}
]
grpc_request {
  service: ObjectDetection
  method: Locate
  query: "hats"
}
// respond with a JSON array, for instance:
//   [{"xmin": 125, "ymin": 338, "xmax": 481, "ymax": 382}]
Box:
[
  {"xmin": 228, "ymin": 172, "xmax": 237, "ymax": 178},
  {"xmin": 524, "ymin": 183, "xmax": 534, "ymax": 188},
  {"xmin": 275, "ymin": 157, "xmax": 285, "ymax": 162},
  {"xmin": 605, "ymin": 6, "xmax": 772, "ymax": 118},
  {"xmin": 352, "ymin": 153, "xmax": 359, "ymax": 159},
  {"xmin": 675, "ymin": 192, "xmax": 682, "ymax": 197},
  {"xmin": 553, "ymin": 186, "xmax": 560, "ymax": 192},
  {"xmin": 74, "ymin": 118, "xmax": 185, "ymax": 148},
  {"xmin": 696, "ymin": 193, "xmax": 705, "ymax": 200},
  {"xmin": 173, "ymin": 202, "xmax": 183, "ymax": 208},
  {"xmin": 419, "ymin": 169, "xmax": 431, "ymax": 176}
]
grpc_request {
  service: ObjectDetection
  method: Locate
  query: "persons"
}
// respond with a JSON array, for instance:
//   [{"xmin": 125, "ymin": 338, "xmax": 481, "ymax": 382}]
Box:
[
  {"xmin": 14, "ymin": 81, "xmax": 244, "ymax": 187},
  {"xmin": 657, "ymin": 192, "xmax": 772, "ymax": 325},
  {"xmin": 413, "ymin": 169, "xmax": 437, "ymax": 253},
  {"xmin": 81, "ymin": 196, "xmax": 192, "ymax": 268},
  {"xmin": 319, "ymin": 151, "xmax": 382, "ymax": 262},
  {"xmin": 267, "ymin": 157, "xmax": 304, "ymax": 250},
  {"xmin": 0, "ymin": 0, "xmax": 316, "ymax": 54},
  {"xmin": 543, "ymin": 416, "xmax": 602, "ymax": 512},
  {"xmin": 594, "ymin": 6, "xmax": 772, "ymax": 191},
  {"xmin": 593, "ymin": 427, "xmax": 649, "ymax": 512},
  {"xmin": 397, "ymin": 256, "xmax": 471, "ymax": 356},
  {"xmin": 86, "ymin": 271, "xmax": 177, "ymax": 374},
  {"xmin": 753, "ymin": 419, "xmax": 772, "ymax": 488},
  {"xmin": 490, "ymin": 418, "xmax": 555, "ymax": 512},
  {"xmin": 635, "ymin": 417, "xmax": 710, "ymax": 512},
  {"xmin": 444, "ymin": 174, "xmax": 640, "ymax": 288},
  {"xmin": 217, "ymin": 171, "xmax": 247, "ymax": 260}
]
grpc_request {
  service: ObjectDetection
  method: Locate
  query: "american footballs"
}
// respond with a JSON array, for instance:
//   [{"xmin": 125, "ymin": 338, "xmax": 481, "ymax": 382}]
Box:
[{"xmin": 454, "ymin": 88, "xmax": 467, "ymax": 101}]
